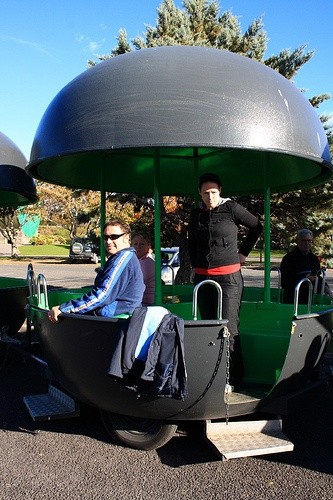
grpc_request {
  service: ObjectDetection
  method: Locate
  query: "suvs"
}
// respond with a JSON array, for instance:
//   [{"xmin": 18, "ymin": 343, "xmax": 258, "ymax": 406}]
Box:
[{"xmin": 68, "ymin": 237, "xmax": 109, "ymax": 264}]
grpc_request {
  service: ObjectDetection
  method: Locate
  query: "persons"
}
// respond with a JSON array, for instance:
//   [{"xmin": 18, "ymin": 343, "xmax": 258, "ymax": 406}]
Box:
[
  {"xmin": 188, "ymin": 174, "xmax": 264, "ymax": 394},
  {"xmin": 131, "ymin": 231, "xmax": 156, "ymax": 307},
  {"xmin": 48, "ymin": 218, "xmax": 144, "ymax": 325},
  {"xmin": 280, "ymin": 229, "xmax": 333, "ymax": 304}
]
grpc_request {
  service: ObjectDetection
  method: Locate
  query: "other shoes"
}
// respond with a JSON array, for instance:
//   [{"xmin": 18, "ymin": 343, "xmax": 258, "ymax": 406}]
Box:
[{"xmin": 225, "ymin": 384, "xmax": 234, "ymax": 393}]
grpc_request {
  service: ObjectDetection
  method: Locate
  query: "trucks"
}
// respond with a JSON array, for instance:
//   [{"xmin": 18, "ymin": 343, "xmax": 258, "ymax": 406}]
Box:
[{"xmin": 0, "ymin": 244, "xmax": 21, "ymax": 257}]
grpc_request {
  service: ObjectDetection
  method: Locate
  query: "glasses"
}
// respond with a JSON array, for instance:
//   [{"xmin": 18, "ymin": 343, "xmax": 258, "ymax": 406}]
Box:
[
  {"xmin": 102, "ymin": 233, "xmax": 126, "ymax": 240},
  {"xmin": 298, "ymin": 240, "xmax": 313, "ymax": 243}
]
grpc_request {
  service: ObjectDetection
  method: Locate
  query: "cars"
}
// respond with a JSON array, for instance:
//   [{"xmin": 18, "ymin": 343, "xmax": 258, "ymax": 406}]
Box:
[
  {"xmin": 159, "ymin": 247, "xmax": 181, "ymax": 286},
  {"xmin": 44, "ymin": 235, "xmax": 67, "ymax": 244}
]
